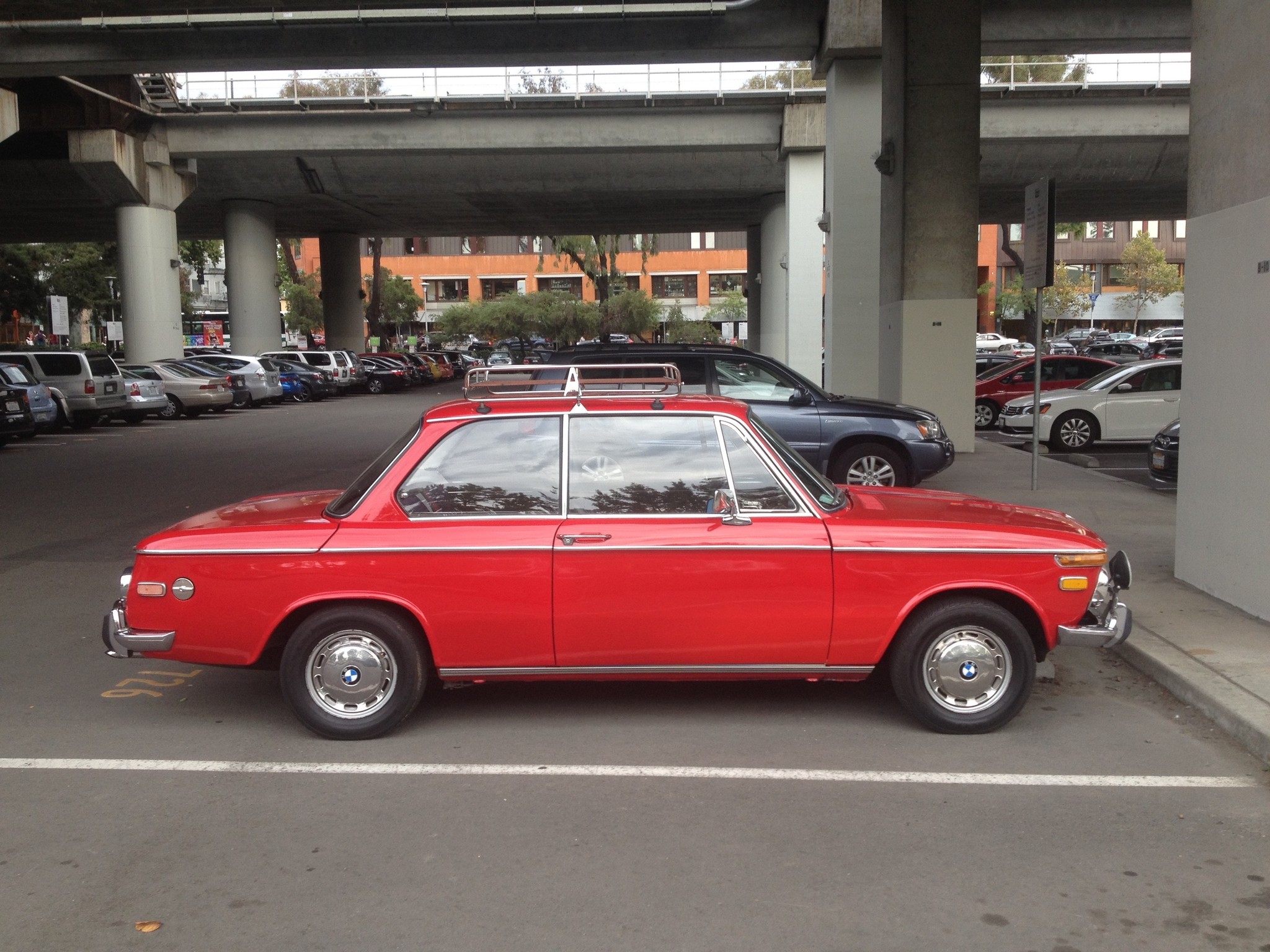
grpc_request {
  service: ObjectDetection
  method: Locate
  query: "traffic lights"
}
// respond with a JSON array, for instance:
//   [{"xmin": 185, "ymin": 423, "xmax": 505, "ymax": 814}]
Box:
[{"xmin": 197, "ymin": 266, "xmax": 205, "ymax": 284}]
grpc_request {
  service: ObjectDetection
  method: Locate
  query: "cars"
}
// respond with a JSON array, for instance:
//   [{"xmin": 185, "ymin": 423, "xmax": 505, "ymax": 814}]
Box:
[
  {"xmin": 298, "ymin": 334, "xmax": 326, "ymax": 351},
  {"xmin": 101, "ymin": 362, "xmax": 1133, "ymax": 735},
  {"xmin": 1148, "ymin": 418, "xmax": 1180, "ymax": 481},
  {"xmin": 998, "ymin": 358, "xmax": 1182, "ymax": 454},
  {"xmin": 108, "ymin": 332, "xmax": 801, "ymax": 463},
  {"xmin": 99, "ymin": 367, "xmax": 169, "ymax": 425},
  {"xmin": 0, "ymin": 362, "xmax": 59, "ymax": 447},
  {"xmin": 975, "ymin": 326, "xmax": 1183, "ymax": 431}
]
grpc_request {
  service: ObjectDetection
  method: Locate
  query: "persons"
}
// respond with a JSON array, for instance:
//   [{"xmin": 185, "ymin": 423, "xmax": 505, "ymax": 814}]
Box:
[
  {"xmin": 26, "ymin": 330, "xmax": 46, "ymax": 347},
  {"xmin": 50, "ymin": 332, "xmax": 70, "ymax": 346},
  {"xmin": 102, "ymin": 336, "xmax": 108, "ymax": 346}
]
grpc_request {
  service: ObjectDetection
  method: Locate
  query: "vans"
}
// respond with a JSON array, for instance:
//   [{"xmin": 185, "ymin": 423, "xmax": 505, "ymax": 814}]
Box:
[{"xmin": 0, "ymin": 350, "xmax": 128, "ymax": 433}]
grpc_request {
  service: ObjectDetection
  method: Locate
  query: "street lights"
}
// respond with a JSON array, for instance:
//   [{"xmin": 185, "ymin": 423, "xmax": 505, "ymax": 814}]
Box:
[
  {"xmin": 419, "ymin": 282, "xmax": 430, "ymax": 333},
  {"xmin": 104, "ymin": 276, "xmax": 117, "ymax": 351},
  {"xmin": 1087, "ymin": 270, "xmax": 1099, "ymax": 328}
]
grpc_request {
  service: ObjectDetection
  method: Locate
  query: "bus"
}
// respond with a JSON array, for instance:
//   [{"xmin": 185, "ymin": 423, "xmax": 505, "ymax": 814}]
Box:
[{"xmin": 182, "ymin": 311, "xmax": 300, "ymax": 352}]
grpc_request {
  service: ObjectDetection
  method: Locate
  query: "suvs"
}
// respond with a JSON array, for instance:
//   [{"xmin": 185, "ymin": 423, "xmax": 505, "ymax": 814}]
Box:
[{"xmin": 525, "ymin": 342, "xmax": 955, "ymax": 487}]
